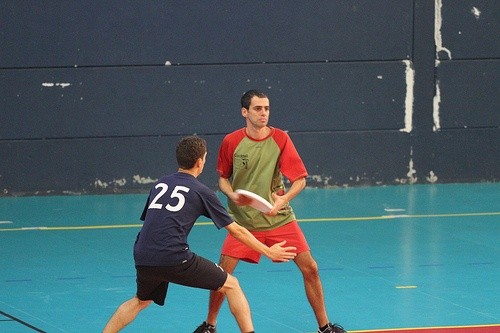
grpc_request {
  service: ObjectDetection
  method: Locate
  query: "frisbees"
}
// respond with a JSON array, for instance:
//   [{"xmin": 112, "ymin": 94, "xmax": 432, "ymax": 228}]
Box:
[{"xmin": 234, "ymin": 189, "xmax": 273, "ymax": 214}]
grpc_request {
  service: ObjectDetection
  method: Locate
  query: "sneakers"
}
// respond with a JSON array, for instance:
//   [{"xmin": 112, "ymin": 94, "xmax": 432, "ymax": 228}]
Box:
[
  {"xmin": 318, "ymin": 323, "xmax": 347, "ymax": 333},
  {"xmin": 193, "ymin": 321, "xmax": 217, "ymax": 333}
]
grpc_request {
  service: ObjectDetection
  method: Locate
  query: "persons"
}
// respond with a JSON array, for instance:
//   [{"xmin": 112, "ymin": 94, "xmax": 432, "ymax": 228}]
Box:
[
  {"xmin": 191, "ymin": 90, "xmax": 348, "ymax": 333},
  {"xmin": 101, "ymin": 135, "xmax": 298, "ymax": 333}
]
